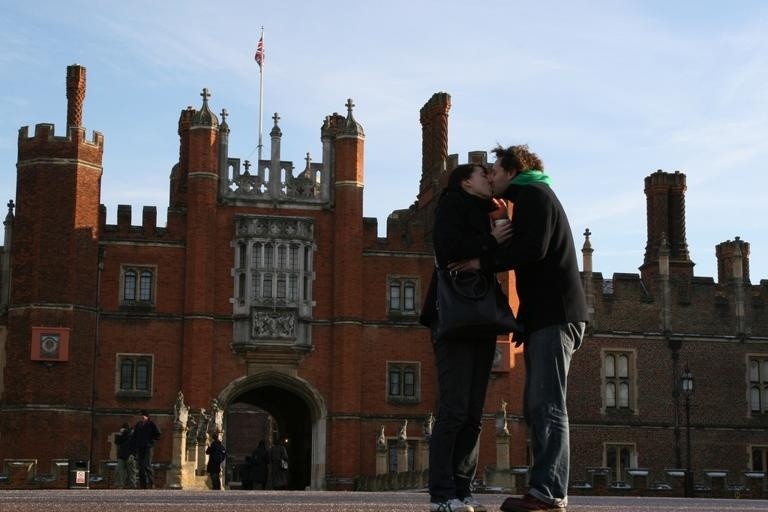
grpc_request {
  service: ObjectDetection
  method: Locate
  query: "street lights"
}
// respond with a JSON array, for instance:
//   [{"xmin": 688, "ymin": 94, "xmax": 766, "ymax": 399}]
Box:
[{"xmin": 681, "ymin": 360, "xmax": 694, "ymax": 498}]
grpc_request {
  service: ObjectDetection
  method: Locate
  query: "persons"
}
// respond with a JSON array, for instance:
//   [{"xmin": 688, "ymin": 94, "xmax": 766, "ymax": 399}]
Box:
[
  {"xmin": 132, "ymin": 410, "xmax": 161, "ymax": 490},
  {"xmin": 250, "ymin": 439, "xmax": 268, "ymax": 490},
  {"xmin": 206, "ymin": 432, "xmax": 225, "ymax": 489},
  {"xmin": 111, "ymin": 422, "xmax": 136, "ymax": 490},
  {"xmin": 446, "ymin": 144, "xmax": 590, "ymax": 511},
  {"xmin": 270, "ymin": 439, "xmax": 289, "ymax": 489},
  {"xmin": 420, "ymin": 162, "xmax": 518, "ymax": 512}
]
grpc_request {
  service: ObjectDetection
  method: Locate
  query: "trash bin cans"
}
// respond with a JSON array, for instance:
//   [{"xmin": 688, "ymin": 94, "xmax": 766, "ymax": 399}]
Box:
[
  {"xmin": 592, "ymin": 473, "xmax": 607, "ymax": 488},
  {"xmin": 68, "ymin": 458, "xmax": 90, "ymax": 489}
]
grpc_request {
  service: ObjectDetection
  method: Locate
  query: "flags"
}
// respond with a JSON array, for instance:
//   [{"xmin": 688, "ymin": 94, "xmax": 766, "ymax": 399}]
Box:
[{"xmin": 254, "ymin": 37, "xmax": 265, "ymax": 68}]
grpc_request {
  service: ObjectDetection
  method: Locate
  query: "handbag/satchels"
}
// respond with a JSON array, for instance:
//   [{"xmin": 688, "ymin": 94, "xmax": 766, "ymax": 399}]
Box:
[{"xmin": 436, "ymin": 269, "xmax": 517, "ymax": 340}]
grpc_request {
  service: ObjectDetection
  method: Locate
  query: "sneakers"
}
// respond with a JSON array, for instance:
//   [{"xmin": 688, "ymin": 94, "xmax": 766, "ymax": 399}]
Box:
[
  {"xmin": 430, "ymin": 497, "xmax": 486, "ymax": 512},
  {"xmin": 500, "ymin": 494, "xmax": 565, "ymax": 512}
]
